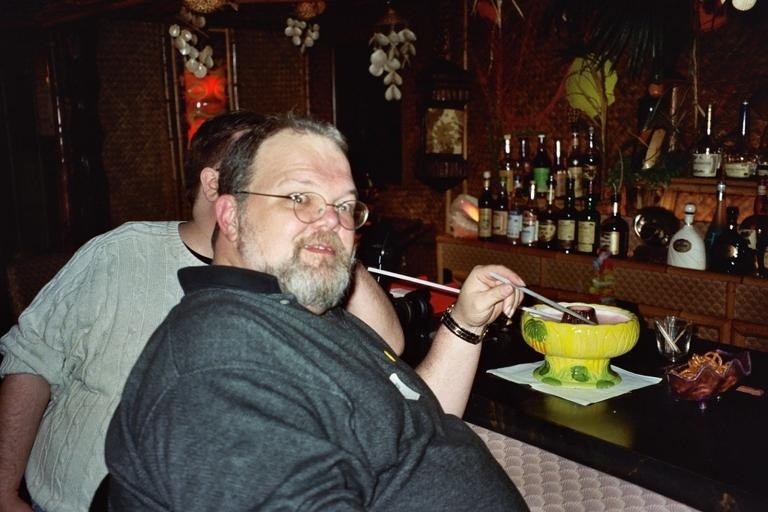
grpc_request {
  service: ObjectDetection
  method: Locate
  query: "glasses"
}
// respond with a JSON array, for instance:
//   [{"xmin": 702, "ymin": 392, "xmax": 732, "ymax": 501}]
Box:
[{"xmin": 231, "ymin": 191, "xmax": 371, "ymax": 231}]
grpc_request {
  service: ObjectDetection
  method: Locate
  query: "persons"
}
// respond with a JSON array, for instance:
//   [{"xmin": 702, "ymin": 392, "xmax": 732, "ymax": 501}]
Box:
[
  {"xmin": 0, "ymin": 113, "xmax": 268, "ymax": 511},
  {"xmin": 105, "ymin": 111, "xmax": 525, "ymax": 512}
]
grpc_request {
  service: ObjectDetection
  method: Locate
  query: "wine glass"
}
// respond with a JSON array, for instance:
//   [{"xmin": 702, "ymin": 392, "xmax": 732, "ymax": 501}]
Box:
[{"xmin": 520, "ymin": 302, "xmax": 640, "ymax": 392}]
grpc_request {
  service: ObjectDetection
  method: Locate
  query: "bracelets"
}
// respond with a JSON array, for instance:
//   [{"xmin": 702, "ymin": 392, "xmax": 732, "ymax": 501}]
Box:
[{"xmin": 441, "ymin": 301, "xmax": 487, "ymax": 345}]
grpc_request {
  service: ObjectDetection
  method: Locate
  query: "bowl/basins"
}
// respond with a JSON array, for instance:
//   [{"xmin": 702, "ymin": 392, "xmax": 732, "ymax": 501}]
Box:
[{"xmin": 657, "ymin": 349, "xmax": 752, "ymax": 410}]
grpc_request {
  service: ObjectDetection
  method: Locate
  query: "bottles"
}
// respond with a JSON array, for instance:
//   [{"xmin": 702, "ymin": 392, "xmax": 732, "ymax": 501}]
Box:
[
  {"xmin": 647, "ymin": 58, "xmax": 663, "ymax": 99},
  {"xmin": 477, "ymin": 101, "xmax": 766, "ymax": 276}
]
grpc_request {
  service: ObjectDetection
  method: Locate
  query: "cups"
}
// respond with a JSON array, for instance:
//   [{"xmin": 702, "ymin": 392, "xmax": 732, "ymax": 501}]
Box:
[{"xmin": 655, "ymin": 317, "xmax": 693, "ymax": 362}]
